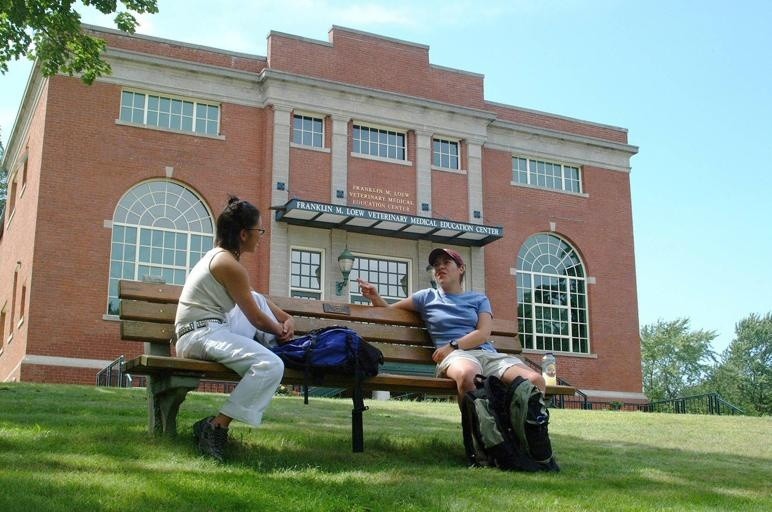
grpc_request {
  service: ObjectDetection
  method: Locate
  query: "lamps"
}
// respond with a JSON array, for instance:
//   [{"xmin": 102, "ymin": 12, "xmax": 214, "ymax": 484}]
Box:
[{"xmin": 335, "ymin": 247, "xmax": 354, "ymax": 295}]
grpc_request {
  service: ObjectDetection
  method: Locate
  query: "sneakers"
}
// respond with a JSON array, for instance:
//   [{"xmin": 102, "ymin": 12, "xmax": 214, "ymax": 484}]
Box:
[{"xmin": 192, "ymin": 413, "xmax": 229, "ymax": 462}]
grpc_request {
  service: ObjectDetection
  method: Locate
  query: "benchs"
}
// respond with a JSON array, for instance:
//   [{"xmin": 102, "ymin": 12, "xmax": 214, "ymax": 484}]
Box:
[{"xmin": 119, "ymin": 280, "xmax": 576, "ymax": 460}]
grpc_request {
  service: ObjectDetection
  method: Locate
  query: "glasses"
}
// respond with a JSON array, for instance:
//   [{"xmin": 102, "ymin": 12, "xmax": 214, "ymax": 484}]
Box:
[{"xmin": 246, "ymin": 227, "xmax": 266, "ymax": 235}]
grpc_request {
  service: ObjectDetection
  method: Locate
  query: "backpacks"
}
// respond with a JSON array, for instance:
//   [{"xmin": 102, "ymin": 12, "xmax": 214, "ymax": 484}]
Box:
[
  {"xmin": 459, "ymin": 372, "xmax": 560, "ymax": 472},
  {"xmin": 269, "ymin": 325, "xmax": 384, "ymax": 386}
]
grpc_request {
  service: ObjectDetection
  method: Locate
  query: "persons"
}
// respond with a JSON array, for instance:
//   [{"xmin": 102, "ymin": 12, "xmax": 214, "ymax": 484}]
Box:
[
  {"xmin": 355, "ymin": 245, "xmax": 560, "ymax": 474},
  {"xmin": 173, "ymin": 193, "xmax": 297, "ymax": 464}
]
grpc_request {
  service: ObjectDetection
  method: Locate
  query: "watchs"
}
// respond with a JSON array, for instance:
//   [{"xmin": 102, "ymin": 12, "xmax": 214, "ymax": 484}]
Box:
[{"xmin": 449, "ymin": 340, "xmax": 459, "ymax": 350}]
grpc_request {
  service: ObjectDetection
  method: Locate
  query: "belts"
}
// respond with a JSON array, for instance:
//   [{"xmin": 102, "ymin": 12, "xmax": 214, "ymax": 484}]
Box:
[{"xmin": 176, "ymin": 318, "xmax": 224, "ymax": 338}]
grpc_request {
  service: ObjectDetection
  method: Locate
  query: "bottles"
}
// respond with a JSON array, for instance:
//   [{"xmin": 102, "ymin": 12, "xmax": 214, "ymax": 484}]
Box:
[{"xmin": 541, "ymin": 352, "xmax": 557, "ymax": 386}]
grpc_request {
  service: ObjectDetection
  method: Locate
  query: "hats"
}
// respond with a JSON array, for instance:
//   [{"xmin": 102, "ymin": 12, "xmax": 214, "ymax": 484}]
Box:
[{"xmin": 428, "ymin": 247, "xmax": 464, "ymax": 268}]
